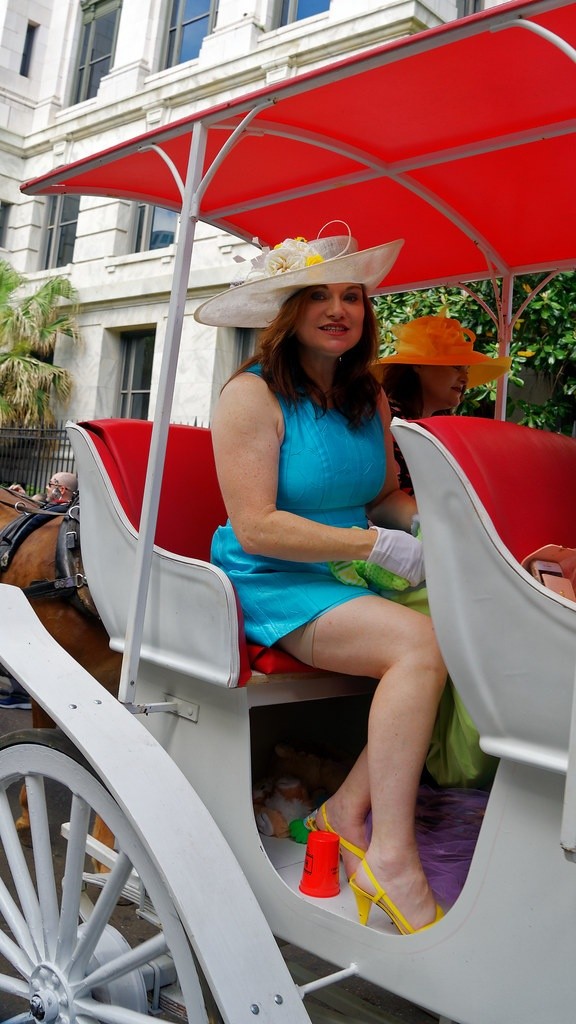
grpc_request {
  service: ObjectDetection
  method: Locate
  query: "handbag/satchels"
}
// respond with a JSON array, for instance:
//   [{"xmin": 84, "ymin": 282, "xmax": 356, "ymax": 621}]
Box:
[{"xmin": 331, "ymin": 517, "xmax": 487, "ymax": 785}]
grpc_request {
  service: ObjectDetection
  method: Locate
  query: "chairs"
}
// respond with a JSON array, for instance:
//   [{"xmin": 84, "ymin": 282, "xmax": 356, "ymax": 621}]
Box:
[{"xmin": 387, "ymin": 416, "xmax": 576, "ymax": 776}]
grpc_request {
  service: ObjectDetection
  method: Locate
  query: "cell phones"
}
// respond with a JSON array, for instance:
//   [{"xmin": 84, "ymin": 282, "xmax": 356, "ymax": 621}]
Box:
[{"xmin": 531, "ymin": 559, "xmax": 565, "ymax": 586}]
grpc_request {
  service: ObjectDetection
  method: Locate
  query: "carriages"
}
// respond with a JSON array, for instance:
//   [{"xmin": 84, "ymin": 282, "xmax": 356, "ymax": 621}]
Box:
[{"xmin": 0, "ymin": 0, "xmax": 576, "ymax": 1024}]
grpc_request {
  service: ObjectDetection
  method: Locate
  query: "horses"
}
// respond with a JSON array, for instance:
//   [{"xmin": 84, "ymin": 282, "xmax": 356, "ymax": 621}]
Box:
[{"xmin": 1, "ymin": 484, "xmax": 123, "ymax": 901}]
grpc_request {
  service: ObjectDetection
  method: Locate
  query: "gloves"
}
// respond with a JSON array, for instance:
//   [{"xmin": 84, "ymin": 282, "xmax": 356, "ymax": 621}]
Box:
[{"xmin": 366, "ymin": 526, "xmax": 426, "ymax": 587}]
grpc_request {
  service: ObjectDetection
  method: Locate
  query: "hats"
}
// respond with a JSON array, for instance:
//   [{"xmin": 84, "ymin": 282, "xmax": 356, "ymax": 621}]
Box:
[
  {"xmin": 193, "ymin": 221, "xmax": 404, "ymax": 329},
  {"xmin": 366, "ymin": 315, "xmax": 512, "ymax": 390}
]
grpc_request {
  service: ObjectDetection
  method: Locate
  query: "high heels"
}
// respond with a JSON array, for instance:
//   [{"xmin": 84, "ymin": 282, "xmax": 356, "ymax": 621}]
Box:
[
  {"xmin": 303, "ymin": 801, "xmax": 366, "ymax": 883},
  {"xmin": 349, "ymin": 857, "xmax": 445, "ymax": 936}
]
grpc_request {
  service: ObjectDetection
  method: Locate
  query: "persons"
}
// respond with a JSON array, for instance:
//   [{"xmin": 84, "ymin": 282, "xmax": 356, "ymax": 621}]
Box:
[
  {"xmin": 9, "ymin": 471, "xmax": 78, "ymax": 507},
  {"xmin": 30, "ymin": 492, "xmax": 47, "ymax": 504},
  {"xmin": 193, "ymin": 227, "xmax": 449, "ymax": 937},
  {"xmin": 370, "ymin": 301, "xmax": 515, "ymax": 789}
]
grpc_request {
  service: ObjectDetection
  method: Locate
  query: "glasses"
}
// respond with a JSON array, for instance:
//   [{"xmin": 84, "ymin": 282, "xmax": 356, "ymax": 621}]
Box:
[
  {"xmin": 444, "ymin": 364, "xmax": 471, "ymax": 375},
  {"xmin": 49, "ymin": 483, "xmax": 69, "ymax": 490}
]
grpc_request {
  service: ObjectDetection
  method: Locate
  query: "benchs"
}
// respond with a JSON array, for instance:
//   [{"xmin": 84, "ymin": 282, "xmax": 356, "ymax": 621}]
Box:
[{"xmin": 64, "ymin": 417, "xmax": 379, "ymax": 708}]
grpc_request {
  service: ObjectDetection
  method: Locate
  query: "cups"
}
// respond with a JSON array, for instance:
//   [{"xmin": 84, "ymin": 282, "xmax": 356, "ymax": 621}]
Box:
[{"xmin": 300, "ymin": 830, "xmax": 343, "ymax": 897}]
